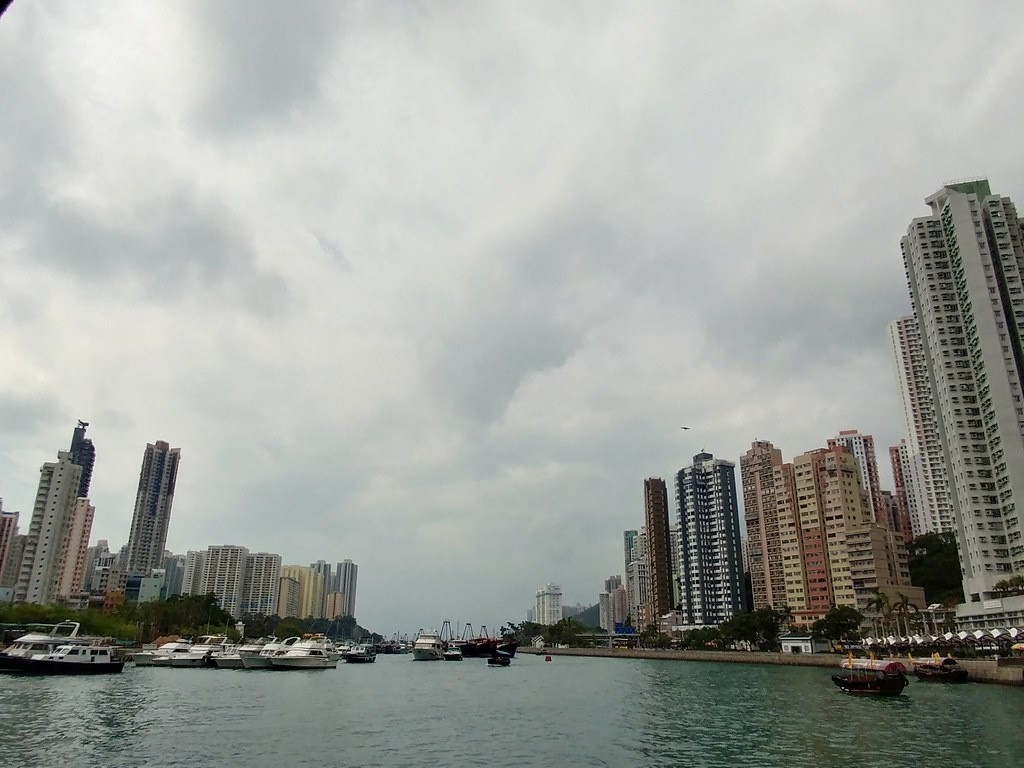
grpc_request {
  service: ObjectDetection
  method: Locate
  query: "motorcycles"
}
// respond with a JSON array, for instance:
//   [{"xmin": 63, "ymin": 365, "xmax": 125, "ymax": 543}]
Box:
[{"xmin": 545, "ymin": 656, "xmax": 552, "ymax": 661}]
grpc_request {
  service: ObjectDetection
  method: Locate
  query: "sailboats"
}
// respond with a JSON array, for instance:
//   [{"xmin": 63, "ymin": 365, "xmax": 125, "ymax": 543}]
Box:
[{"xmin": 375, "ymin": 620, "xmax": 502, "ymax": 658}]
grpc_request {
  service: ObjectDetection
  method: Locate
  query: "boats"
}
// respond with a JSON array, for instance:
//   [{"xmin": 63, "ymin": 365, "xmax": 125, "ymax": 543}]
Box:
[
  {"xmin": 0, "ymin": 620, "xmax": 126, "ymax": 678},
  {"xmin": 831, "ymin": 673, "xmax": 909, "ymax": 696},
  {"xmin": 488, "ymin": 656, "xmax": 511, "ymax": 667},
  {"xmin": 912, "ymin": 657, "xmax": 969, "ymax": 682},
  {"xmin": 258, "ymin": 632, "xmax": 343, "ymax": 669},
  {"xmin": 443, "ymin": 647, "xmax": 462, "ymax": 661},
  {"xmin": 497, "ymin": 633, "xmax": 519, "ymax": 659},
  {"xmin": 335, "ymin": 637, "xmax": 377, "ymax": 663},
  {"xmin": 412, "ymin": 633, "xmax": 445, "ymax": 660},
  {"xmin": 125, "ymin": 635, "xmax": 272, "ymax": 670}
]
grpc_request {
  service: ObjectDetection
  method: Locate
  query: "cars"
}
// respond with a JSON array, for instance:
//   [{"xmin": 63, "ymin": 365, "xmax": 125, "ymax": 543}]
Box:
[{"xmin": 535, "ymin": 649, "xmax": 547, "ymax": 655}]
